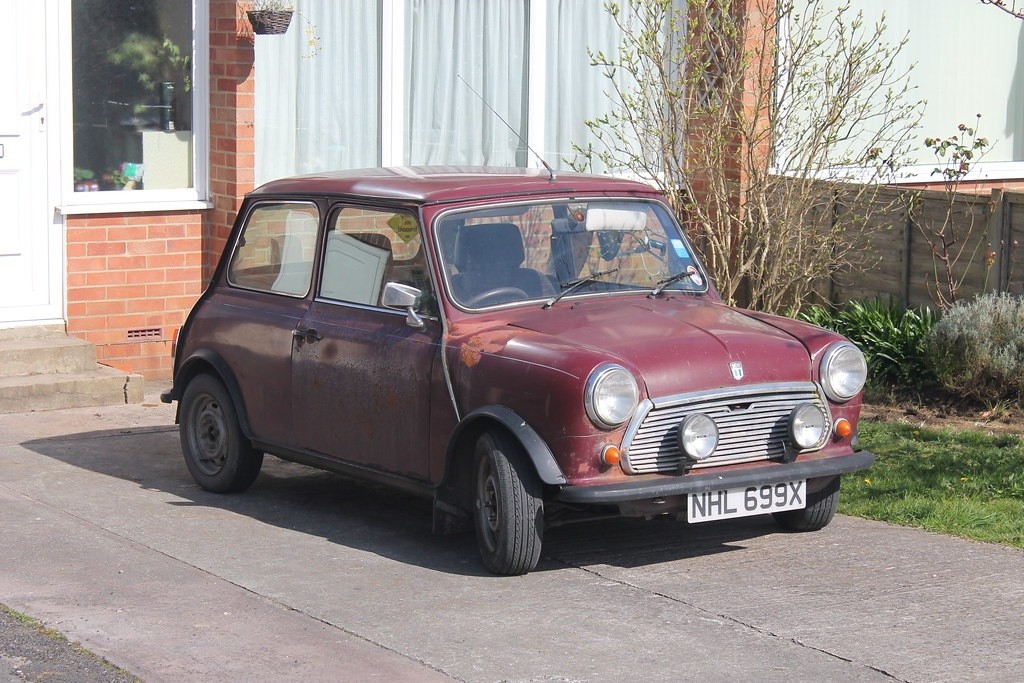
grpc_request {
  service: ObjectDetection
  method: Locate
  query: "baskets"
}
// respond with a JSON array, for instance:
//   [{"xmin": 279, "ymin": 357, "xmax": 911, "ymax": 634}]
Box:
[{"xmin": 245, "ymin": 10, "xmax": 293, "ymax": 34}]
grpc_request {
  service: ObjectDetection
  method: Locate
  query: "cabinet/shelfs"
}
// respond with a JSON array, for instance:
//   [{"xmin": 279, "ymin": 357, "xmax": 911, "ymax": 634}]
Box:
[{"xmin": 108, "ymin": 126, "xmax": 192, "ymax": 193}]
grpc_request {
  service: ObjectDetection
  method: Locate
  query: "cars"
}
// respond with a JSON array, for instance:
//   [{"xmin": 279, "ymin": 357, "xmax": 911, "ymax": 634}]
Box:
[{"xmin": 160, "ymin": 165, "xmax": 874, "ymax": 576}]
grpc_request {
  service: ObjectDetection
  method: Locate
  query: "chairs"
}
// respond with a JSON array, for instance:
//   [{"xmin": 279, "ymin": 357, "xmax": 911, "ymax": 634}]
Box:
[
  {"xmin": 332, "ymin": 233, "xmax": 393, "ymax": 308},
  {"xmin": 449, "ymin": 221, "xmax": 556, "ymax": 301}
]
